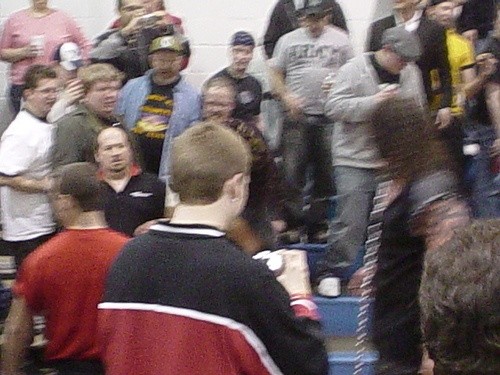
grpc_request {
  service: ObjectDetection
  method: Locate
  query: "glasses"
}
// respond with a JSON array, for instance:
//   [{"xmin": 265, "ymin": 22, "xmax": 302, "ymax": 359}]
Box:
[{"xmin": 32, "ymin": 87, "xmax": 59, "ymax": 95}]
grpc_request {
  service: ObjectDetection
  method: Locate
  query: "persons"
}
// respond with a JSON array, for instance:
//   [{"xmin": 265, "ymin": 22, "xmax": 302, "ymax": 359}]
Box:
[
  {"xmin": 1, "ymin": 162, "xmax": 134, "ymax": 374},
  {"xmin": 350, "ymin": 95, "xmax": 470, "ymax": 375},
  {"xmin": 319, "ymin": 28, "xmax": 428, "ymax": 299},
  {"xmin": 199, "ymin": 31, "xmax": 270, "ymax": 144},
  {"xmin": 1, "ymin": 66, "xmax": 58, "ymax": 334},
  {"xmin": 96, "ymin": 127, "xmax": 164, "ymax": 239},
  {"xmin": 271, "ymin": 0, "xmax": 358, "ymax": 225},
  {"xmin": 98, "ymin": 123, "xmax": 328, "ymax": 375},
  {"xmin": 367, "ymin": 0, "xmax": 500, "ymax": 129},
  {"xmin": 264, "ymin": 0, "xmax": 350, "ymax": 62},
  {"xmin": 459, "ymin": 55, "xmax": 500, "ymax": 220},
  {"xmin": 422, "ymin": 220, "xmax": 500, "ymax": 375},
  {"xmin": 0, "ymin": 0, "xmax": 190, "ymax": 122},
  {"xmin": 116, "ymin": 32, "xmax": 199, "ymax": 179},
  {"xmin": 52, "ymin": 64, "xmax": 143, "ymax": 229},
  {"xmin": 187, "ymin": 79, "xmax": 274, "ymax": 245}
]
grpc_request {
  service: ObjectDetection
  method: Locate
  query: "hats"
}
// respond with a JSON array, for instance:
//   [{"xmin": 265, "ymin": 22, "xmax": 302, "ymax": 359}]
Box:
[
  {"xmin": 295, "ymin": 0, "xmax": 334, "ymax": 18},
  {"xmin": 147, "ymin": 36, "xmax": 182, "ymax": 55},
  {"xmin": 229, "ymin": 30, "xmax": 255, "ymax": 49},
  {"xmin": 380, "ymin": 27, "xmax": 421, "ymax": 62}
]
